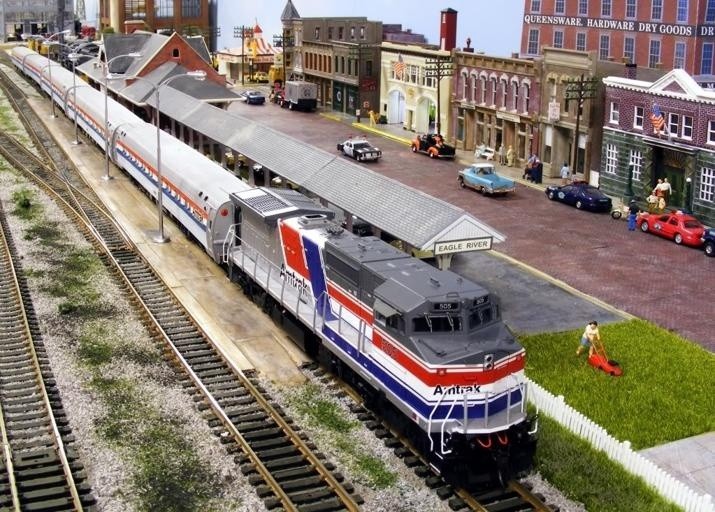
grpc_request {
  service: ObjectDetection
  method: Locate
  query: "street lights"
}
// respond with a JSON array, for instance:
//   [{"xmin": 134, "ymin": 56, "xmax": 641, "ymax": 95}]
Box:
[
  {"xmin": 425, "ymin": 56, "xmax": 455, "ymax": 134},
  {"xmin": 234, "ymin": 26, "xmax": 254, "ymax": 85},
  {"xmin": 348, "ymin": 42, "xmax": 374, "ymax": 123},
  {"xmin": 20, "ymin": 29, "xmax": 207, "ymax": 243},
  {"xmin": 561, "ymin": 73, "xmax": 599, "ymax": 183},
  {"xmin": 273, "ymin": 33, "xmax": 296, "ymax": 80}
]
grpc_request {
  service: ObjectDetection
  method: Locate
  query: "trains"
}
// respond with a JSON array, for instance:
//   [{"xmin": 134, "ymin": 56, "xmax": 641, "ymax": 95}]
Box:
[{"xmin": 11, "ymin": 46, "xmax": 541, "ymax": 491}]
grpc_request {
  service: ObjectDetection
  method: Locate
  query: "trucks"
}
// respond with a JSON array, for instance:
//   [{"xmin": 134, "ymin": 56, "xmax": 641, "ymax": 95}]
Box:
[
  {"xmin": 269, "ymin": 64, "xmax": 293, "ymax": 87},
  {"xmin": 277, "ymin": 81, "xmax": 317, "ymax": 109}
]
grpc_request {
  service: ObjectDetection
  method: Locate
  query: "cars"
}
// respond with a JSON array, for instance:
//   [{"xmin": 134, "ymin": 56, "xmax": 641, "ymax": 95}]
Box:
[
  {"xmin": 457, "ymin": 163, "xmax": 516, "ymax": 196},
  {"xmin": 412, "ymin": 134, "xmax": 455, "ymax": 157},
  {"xmin": 636, "ymin": 208, "xmax": 715, "ymax": 256},
  {"xmin": 336, "ymin": 134, "xmax": 382, "ymax": 162},
  {"xmin": 240, "ymin": 90, "xmax": 265, "ymax": 104},
  {"xmin": 247, "ymin": 71, "xmax": 269, "ymax": 83},
  {"xmin": 545, "ymin": 181, "xmax": 613, "ymax": 210}
]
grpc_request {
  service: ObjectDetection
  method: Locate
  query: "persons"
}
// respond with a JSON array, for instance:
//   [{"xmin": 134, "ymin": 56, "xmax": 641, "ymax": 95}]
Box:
[
  {"xmin": 646, "ymin": 177, "xmax": 672, "ymax": 210},
  {"xmin": 524, "ymin": 152, "xmax": 543, "ymax": 184},
  {"xmin": 355, "ymin": 107, "xmax": 361, "ymax": 123},
  {"xmin": 628, "ymin": 199, "xmax": 641, "ymax": 230},
  {"xmin": 475, "ymin": 141, "xmax": 486, "ymax": 159},
  {"xmin": 577, "ymin": 320, "xmax": 602, "ymax": 359},
  {"xmin": 424, "ymin": 133, "xmax": 442, "ymax": 151},
  {"xmin": 507, "ymin": 145, "xmax": 514, "ymax": 167},
  {"xmin": 367, "ymin": 108, "xmax": 380, "ymax": 127},
  {"xmin": 561, "ymin": 162, "xmax": 569, "ymax": 186}
]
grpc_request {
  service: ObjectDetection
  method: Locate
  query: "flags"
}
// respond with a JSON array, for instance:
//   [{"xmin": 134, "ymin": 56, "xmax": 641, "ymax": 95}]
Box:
[
  {"xmin": 652, "ymin": 102, "xmax": 664, "ymax": 131},
  {"xmin": 394, "ymin": 54, "xmax": 405, "ymax": 78}
]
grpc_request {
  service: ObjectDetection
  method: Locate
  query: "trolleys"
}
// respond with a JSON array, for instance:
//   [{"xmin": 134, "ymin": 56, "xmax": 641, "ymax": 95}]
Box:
[{"xmin": 591, "ymin": 339, "xmax": 623, "ymax": 376}]
